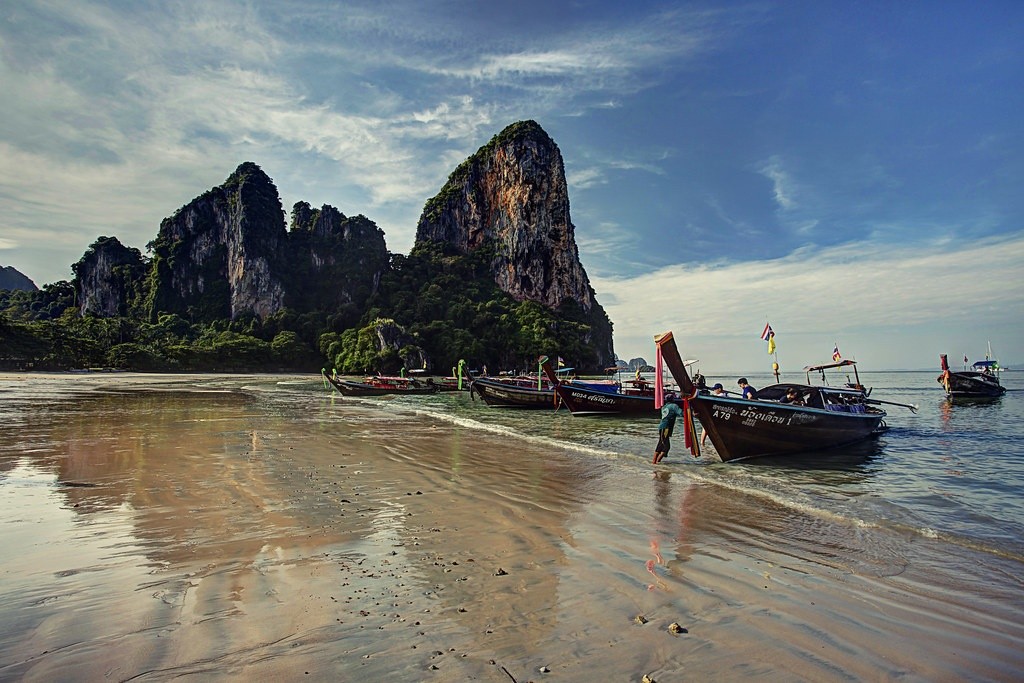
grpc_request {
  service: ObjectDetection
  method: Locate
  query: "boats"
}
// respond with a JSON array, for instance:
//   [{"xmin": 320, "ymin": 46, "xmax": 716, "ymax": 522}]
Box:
[
  {"xmin": 538, "ymin": 355, "xmax": 699, "ymax": 416},
  {"xmin": 321, "ymin": 366, "xmax": 576, "ymax": 397},
  {"xmin": 654, "ymin": 331, "xmax": 887, "ymax": 460},
  {"xmin": 937, "ymin": 340, "xmax": 1007, "ymax": 405},
  {"xmin": 458, "ymin": 359, "xmax": 568, "ymax": 408}
]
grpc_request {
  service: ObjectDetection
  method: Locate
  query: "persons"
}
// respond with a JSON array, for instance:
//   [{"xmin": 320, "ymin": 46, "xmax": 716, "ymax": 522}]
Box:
[
  {"xmin": 701, "ymin": 383, "xmax": 724, "ymax": 445},
  {"xmin": 807, "ymin": 392, "xmax": 855, "ymax": 404},
  {"xmin": 633, "ymin": 376, "xmax": 646, "ymax": 388},
  {"xmin": 651, "ymin": 394, "xmax": 698, "ymax": 464},
  {"xmin": 780, "ymin": 387, "xmax": 805, "ymax": 405},
  {"xmin": 738, "ymin": 378, "xmax": 758, "ymax": 400},
  {"xmin": 983, "ymin": 366, "xmax": 991, "ymax": 375}
]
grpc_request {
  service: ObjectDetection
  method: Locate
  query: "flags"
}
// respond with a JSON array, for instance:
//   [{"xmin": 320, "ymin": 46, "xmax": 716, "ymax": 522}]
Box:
[
  {"xmin": 833, "ymin": 347, "xmax": 842, "ymax": 372},
  {"xmin": 559, "ymin": 358, "xmax": 564, "ymax": 366},
  {"xmin": 761, "ymin": 324, "xmax": 776, "ymax": 355}
]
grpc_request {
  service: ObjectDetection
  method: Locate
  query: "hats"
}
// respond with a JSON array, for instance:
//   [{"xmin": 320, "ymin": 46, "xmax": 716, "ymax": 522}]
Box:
[
  {"xmin": 712, "ymin": 383, "xmax": 723, "ymax": 388},
  {"xmin": 665, "ymin": 394, "xmax": 674, "ymax": 400}
]
